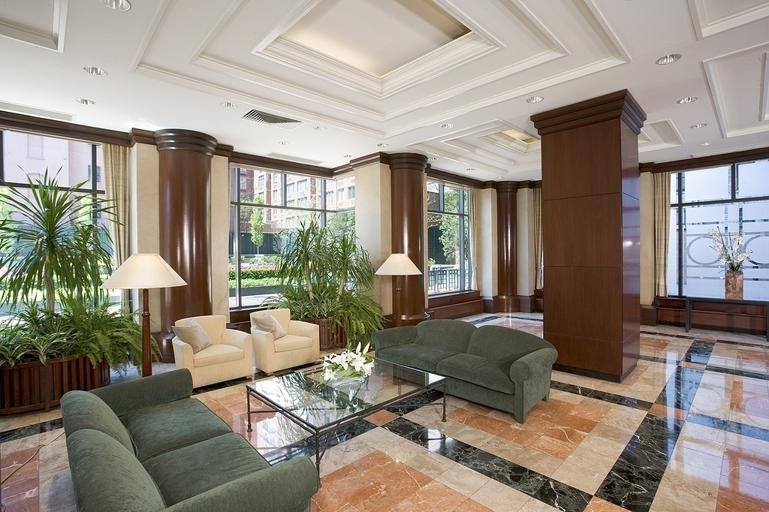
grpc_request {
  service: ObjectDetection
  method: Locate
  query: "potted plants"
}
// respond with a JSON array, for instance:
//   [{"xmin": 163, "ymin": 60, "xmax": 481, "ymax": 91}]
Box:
[
  {"xmin": 0, "ymin": 160, "xmax": 161, "ymax": 415},
  {"xmin": 267, "ymin": 213, "xmax": 390, "ymax": 350}
]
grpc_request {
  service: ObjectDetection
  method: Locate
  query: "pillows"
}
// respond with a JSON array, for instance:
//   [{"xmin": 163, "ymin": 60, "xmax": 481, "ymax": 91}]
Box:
[
  {"xmin": 251, "ymin": 311, "xmax": 286, "ymax": 342},
  {"xmin": 170, "ymin": 320, "xmax": 212, "ymax": 354}
]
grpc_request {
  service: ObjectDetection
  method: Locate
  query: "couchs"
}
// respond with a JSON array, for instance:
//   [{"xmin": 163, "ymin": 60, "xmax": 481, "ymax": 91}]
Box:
[
  {"xmin": 375, "ymin": 316, "xmax": 557, "ymax": 423},
  {"xmin": 58, "ymin": 365, "xmax": 321, "ymax": 512}
]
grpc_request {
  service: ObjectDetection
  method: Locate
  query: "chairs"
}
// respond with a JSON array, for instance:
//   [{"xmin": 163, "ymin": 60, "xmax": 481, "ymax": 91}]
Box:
[
  {"xmin": 170, "ymin": 314, "xmax": 254, "ymax": 396},
  {"xmin": 248, "ymin": 307, "xmax": 319, "ymax": 378}
]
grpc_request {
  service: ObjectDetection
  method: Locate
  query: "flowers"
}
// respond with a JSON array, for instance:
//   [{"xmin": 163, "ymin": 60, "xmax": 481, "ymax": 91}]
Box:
[
  {"xmin": 319, "ymin": 337, "xmax": 377, "ymax": 385},
  {"xmin": 705, "ymin": 224, "xmax": 752, "ymax": 278}
]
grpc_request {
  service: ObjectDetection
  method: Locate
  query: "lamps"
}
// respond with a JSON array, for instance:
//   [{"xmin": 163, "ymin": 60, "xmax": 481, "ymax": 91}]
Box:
[
  {"xmin": 94, "ymin": 251, "xmax": 188, "ymax": 378},
  {"xmin": 373, "ymin": 252, "xmax": 423, "ymax": 328}
]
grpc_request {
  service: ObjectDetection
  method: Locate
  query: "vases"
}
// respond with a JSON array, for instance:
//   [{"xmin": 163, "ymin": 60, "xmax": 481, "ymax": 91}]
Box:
[{"xmin": 725, "ymin": 269, "xmax": 744, "ymax": 301}]
